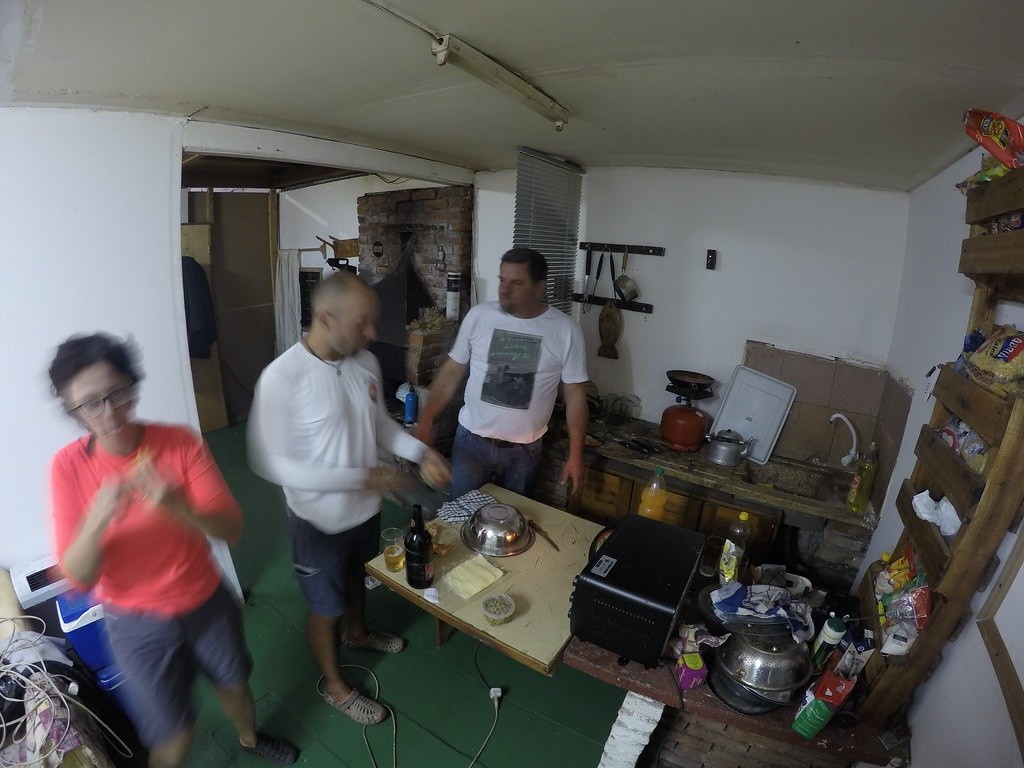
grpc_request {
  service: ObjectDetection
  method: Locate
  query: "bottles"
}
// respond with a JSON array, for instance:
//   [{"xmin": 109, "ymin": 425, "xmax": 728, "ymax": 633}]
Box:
[
  {"xmin": 810, "ymin": 617, "xmax": 848, "ymax": 679},
  {"xmin": 638, "ymin": 468, "xmax": 668, "ymax": 519},
  {"xmin": 842, "ymin": 441, "xmax": 880, "ymax": 512},
  {"xmin": 404, "ymin": 504, "xmax": 434, "ymax": 589},
  {"xmin": 859, "ymin": 552, "xmax": 891, "ymax": 598},
  {"xmin": 718, "ymin": 511, "xmax": 750, "ymax": 577}
]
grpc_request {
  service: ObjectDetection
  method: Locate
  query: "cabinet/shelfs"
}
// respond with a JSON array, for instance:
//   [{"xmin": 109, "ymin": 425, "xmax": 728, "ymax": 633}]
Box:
[{"xmin": 529, "ymin": 455, "xmax": 784, "ymax": 557}]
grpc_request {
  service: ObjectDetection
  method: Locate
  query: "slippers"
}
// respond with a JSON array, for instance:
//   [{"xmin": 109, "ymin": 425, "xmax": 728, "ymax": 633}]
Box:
[
  {"xmin": 238, "ymin": 733, "xmax": 297, "ymax": 764},
  {"xmin": 342, "ymin": 630, "xmax": 403, "ymax": 653},
  {"xmin": 323, "ymin": 687, "xmax": 386, "ymax": 725}
]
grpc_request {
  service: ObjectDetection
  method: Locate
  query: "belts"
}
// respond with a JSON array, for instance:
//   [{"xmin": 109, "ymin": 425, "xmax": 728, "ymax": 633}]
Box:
[{"xmin": 476, "ymin": 436, "xmax": 515, "ymax": 448}]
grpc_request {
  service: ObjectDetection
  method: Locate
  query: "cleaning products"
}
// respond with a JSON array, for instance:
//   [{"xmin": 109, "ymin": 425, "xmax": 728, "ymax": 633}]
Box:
[{"xmin": 845, "ymin": 441, "xmax": 878, "ymax": 514}]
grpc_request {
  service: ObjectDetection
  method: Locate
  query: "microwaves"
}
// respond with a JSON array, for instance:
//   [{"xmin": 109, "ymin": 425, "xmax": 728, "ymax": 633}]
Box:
[{"xmin": 568, "ymin": 513, "xmax": 707, "ymax": 666}]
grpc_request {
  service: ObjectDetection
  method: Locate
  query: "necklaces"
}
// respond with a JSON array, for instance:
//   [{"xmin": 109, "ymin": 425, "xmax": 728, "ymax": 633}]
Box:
[{"xmin": 304, "ymin": 340, "xmax": 346, "ymax": 377}]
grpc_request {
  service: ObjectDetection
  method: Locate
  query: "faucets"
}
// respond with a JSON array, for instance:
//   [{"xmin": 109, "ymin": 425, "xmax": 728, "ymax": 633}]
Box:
[{"xmin": 827, "ymin": 412, "xmax": 859, "ymax": 467}]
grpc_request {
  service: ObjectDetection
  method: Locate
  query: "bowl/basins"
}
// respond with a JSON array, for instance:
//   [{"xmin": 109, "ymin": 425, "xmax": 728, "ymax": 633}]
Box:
[
  {"xmin": 460, "ymin": 503, "xmax": 536, "ymax": 557},
  {"xmin": 704, "ymin": 624, "xmax": 814, "ymax": 717}
]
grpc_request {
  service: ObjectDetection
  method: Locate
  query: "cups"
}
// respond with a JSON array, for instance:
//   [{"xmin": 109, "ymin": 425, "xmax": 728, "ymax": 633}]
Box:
[
  {"xmin": 700, "ymin": 537, "xmax": 728, "ymax": 576},
  {"xmin": 381, "ymin": 527, "xmax": 407, "ymax": 573}
]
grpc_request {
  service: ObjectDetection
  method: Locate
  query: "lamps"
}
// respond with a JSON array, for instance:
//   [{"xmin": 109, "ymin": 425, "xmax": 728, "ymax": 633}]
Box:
[{"xmin": 431, "ymin": 34, "xmax": 570, "ymax": 133}]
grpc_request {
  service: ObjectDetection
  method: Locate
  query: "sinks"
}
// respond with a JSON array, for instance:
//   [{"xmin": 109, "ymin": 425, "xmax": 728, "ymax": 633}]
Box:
[{"xmin": 741, "ymin": 454, "xmax": 833, "ymax": 503}]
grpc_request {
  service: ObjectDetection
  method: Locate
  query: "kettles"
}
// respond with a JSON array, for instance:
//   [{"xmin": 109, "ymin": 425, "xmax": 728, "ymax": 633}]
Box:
[{"xmin": 703, "ymin": 428, "xmax": 754, "ymax": 467}]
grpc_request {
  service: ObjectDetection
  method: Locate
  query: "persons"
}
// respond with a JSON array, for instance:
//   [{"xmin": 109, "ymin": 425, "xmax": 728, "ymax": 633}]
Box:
[
  {"xmin": 416, "ymin": 249, "xmax": 590, "ymax": 502},
  {"xmin": 47, "ymin": 332, "xmax": 300, "ymax": 767},
  {"xmin": 244, "ymin": 272, "xmax": 455, "ymax": 724}
]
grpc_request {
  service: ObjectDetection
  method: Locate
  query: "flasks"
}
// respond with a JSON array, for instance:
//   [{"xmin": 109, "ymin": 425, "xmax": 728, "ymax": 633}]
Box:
[{"xmin": 404, "ymin": 387, "xmax": 419, "ymax": 423}]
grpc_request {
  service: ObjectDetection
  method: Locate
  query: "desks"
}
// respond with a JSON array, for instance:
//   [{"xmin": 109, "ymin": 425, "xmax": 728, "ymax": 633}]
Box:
[
  {"xmin": 563, "ymin": 598, "xmax": 910, "ymax": 766},
  {"xmin": 365, "ymin": 483, "xmax": 613, "ymax": 677}
]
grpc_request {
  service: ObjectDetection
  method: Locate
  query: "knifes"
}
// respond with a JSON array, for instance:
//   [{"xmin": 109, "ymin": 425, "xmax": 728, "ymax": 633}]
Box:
[{"xmin": 528, "ymin": 519, "xmax": 561, "ymax": 552}]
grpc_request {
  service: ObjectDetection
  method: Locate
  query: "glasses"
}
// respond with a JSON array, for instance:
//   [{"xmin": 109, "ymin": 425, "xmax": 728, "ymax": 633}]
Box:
[{"xmin": 64, "ymin": 379, "xmax": 133, "ymax": 421}]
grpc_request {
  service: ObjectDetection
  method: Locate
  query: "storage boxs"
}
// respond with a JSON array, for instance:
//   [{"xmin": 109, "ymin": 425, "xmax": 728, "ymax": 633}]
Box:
[
  {"xmin": 55, "ymin": 589, "xmax": 117, "ymax": 672},
  {"xmin": 96, "ymin": 663, "xmax": 195, "ymax": 718}
]
